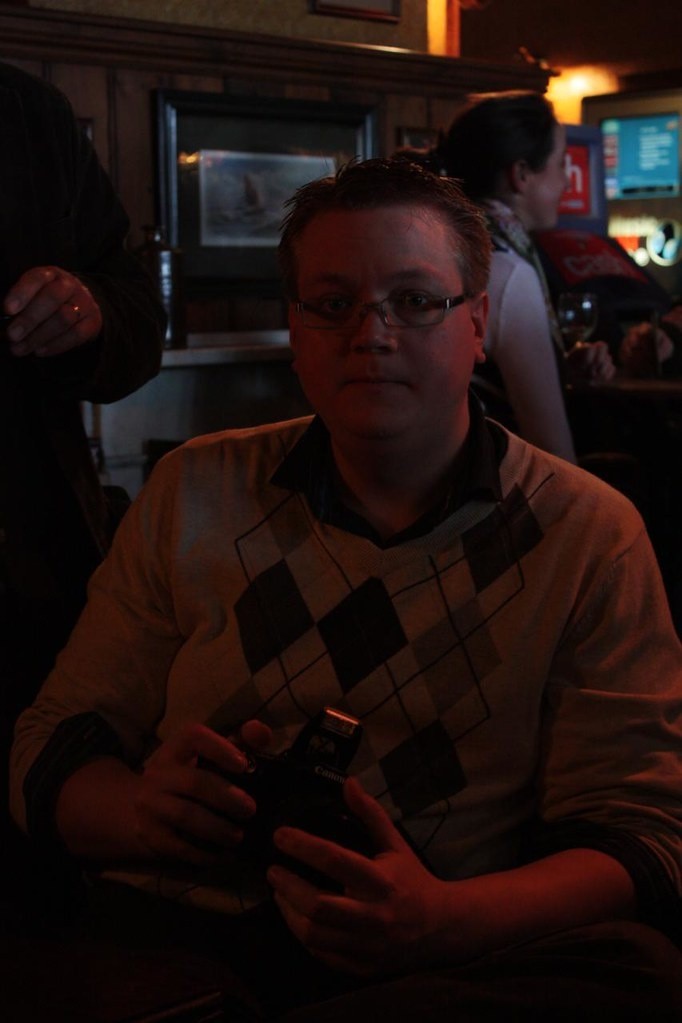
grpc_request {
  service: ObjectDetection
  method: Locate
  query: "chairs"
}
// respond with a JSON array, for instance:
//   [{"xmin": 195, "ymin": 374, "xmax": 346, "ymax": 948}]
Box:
[{"xmin": 470, "ymin": 375, "xmax": 643, "ymax": 506}]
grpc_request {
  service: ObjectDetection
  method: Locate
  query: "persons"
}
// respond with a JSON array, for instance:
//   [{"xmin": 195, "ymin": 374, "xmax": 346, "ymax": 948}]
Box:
[
  {"xmin": 426, "ymin": 89, "xmax": 614, "ymax": 471},
  {"xmin": 8, "ymin": 153, "xmax": 682, "ymax": 1023},
  {"xmin": 619, "ymin": 305, "xmax": 682, "ymax": 378},
  {"xmin": 0, "ymin": 65, "xmax": 170, "ymax": 1023}
]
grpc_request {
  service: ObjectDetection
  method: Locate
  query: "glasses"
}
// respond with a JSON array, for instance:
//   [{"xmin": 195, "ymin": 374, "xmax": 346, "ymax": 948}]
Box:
[{"xmin": 288, "ymin": 289, "xmax": 474, "ymax": 329}]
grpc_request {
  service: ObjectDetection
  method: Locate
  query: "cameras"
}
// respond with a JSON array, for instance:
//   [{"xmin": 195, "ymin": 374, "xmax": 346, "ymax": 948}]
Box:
[{"xmin": 190, "ymin": 706, "xmax": 385, "ymax": 898}]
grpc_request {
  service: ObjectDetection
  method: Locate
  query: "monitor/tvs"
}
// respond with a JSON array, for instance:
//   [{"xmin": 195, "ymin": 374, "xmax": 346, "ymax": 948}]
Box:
[{"xmin": 599, "ymin": 113, "xmax": 682, "ymax": 200}]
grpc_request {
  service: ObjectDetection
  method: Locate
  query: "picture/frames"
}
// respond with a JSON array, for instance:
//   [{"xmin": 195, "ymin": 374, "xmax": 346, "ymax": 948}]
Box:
[
  {"xmin": 312, "ymin": 0, "xmax": 401, "ymax": 24},
  {"xmin": 149, "ymin": 86, "xmax": 384, "ymax": 298}
]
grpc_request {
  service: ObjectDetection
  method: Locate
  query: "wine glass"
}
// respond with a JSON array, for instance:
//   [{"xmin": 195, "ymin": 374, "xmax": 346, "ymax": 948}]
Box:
[{"xmin": 557, "ymin": 292, "xmax": 599, "ymax": 360}]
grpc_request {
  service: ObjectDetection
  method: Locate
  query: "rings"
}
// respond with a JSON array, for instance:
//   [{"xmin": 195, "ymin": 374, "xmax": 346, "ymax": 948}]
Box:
[{"xmin": 66, "ymin": 301, "xmax": 82, "ymax": 320}]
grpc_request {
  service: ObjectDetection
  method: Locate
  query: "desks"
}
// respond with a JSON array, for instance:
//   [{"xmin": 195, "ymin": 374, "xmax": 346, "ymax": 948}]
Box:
[{"xmin": 593, "ymin": 377, "xmax": 682, "ymax": 403}]
